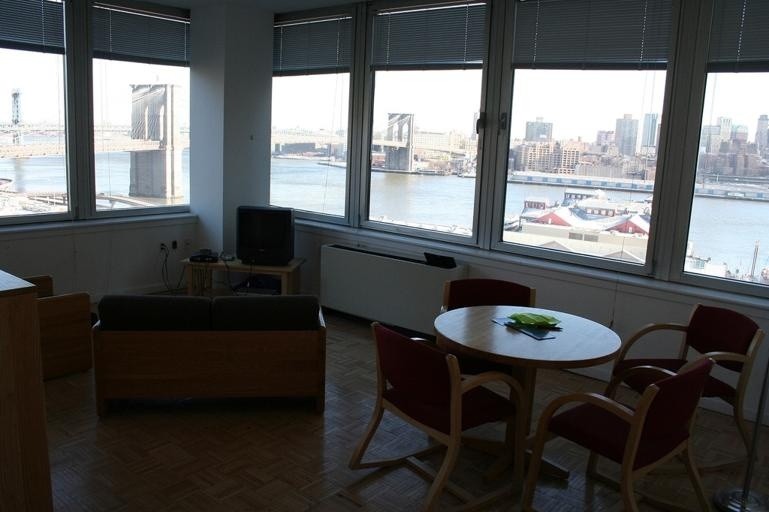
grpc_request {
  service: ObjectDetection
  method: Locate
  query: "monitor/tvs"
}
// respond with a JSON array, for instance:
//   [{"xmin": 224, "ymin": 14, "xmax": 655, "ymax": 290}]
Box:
[{"xmin": 236, "ymin": 205, "xmax": 295, "ymax": 267}]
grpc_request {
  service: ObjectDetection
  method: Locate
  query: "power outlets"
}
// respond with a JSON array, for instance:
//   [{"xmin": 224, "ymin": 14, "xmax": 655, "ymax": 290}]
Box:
[
  {"xmin": 169, "ymin": 240, "xmax": 179, "ymax": 248},
  {"xmin": 160, "ymin": 240, "xmax": 167, "ymax": 250}
]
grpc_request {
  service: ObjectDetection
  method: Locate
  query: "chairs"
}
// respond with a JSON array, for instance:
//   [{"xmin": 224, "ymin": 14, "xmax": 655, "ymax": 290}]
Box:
[
  {"xmin": 517, "ymin": 355, "xmax": 716, "ymax": 512},
  {"xmin": 583, "ymin": 301, "xmax": 766, "ymax": 478},
  {"xmin": 347, "ymin": 320, "xmax": 530, "ymax": 509},
  {"xmin": 434, "ymin": 278, "xmax": 536, "ymax": 383},
  {"xmin": 19, "ymin": 273, "xmax": 92, "ymax": 382}
]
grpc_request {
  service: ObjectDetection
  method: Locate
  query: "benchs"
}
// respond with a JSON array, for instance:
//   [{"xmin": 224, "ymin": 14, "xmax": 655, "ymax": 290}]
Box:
[{"xmin": 92, "ymin": 293, "xmax": 329, "ymax": 422}]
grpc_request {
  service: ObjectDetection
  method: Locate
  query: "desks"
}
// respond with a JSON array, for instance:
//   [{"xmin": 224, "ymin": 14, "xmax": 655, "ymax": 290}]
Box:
[{"xmin": 429, "ymin": 305, "xmax": 622, "ymax": 482}]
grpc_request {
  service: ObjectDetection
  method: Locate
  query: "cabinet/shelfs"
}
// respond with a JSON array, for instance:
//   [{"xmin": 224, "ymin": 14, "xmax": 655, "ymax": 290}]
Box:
[
  {"xmin": 181, "ymin": 252, "xmax": 307, "ymax": 297},
  {"xmin": 0, "ymin": 267, "xmax": 56, "ymax": 512}
]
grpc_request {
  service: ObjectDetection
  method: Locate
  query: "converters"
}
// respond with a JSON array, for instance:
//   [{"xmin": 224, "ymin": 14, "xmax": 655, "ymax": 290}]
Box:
[{"xmin": 172, "ymin": 241, "xmax": 177, "ymax": 249}]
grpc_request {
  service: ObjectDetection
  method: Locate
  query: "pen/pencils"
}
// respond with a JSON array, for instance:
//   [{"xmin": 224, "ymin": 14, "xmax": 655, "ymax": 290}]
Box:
[{"xmin": 508, "ymin": 322, "xmax": 563, "ymax": 330}]
